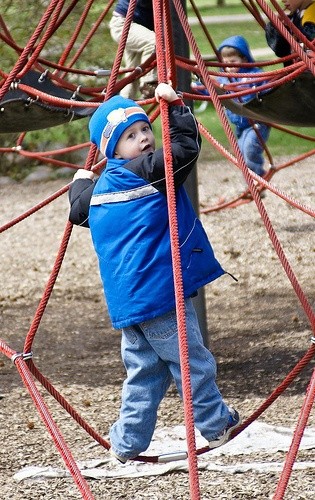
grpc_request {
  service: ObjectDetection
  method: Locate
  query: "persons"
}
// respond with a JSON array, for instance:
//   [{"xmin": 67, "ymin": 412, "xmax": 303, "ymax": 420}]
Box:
[
  {"xmin": 191, "ymin": 36, "xmax": 271, "ymax": 201},
  {"xmin": 109, "ymin": 0, "xmax": 160, "ymax": 101},
  {"xmin": 70, "ymin": 84, "xmax": 243, "ymax": 463},
  {"xmin": 265, "ymin": 0, "xmax": 315, "ymax": 66}
]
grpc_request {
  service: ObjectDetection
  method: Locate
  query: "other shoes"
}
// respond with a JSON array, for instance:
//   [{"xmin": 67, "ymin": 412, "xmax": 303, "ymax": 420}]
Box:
[
  {"xmin": 140, "ymin": 81, "xmax": 183, "ymax": 98},
  {"xmin": 239, "ymin": 191, "xmax": 266, "ymax": 198}
]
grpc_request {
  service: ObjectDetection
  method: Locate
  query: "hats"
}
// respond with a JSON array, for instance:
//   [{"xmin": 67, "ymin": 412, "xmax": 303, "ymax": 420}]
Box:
[{"xmin": 88, "ymin": 95, "xmax": 152, "ymax": 159}]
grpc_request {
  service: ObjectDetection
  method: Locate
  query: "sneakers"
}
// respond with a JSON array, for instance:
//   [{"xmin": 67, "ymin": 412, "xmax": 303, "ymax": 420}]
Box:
[
  {"xmin": 210, "ymin": 406, "xmax": 241, "ymax": 448},
  {"xmin": 109, "ymin": 447, "xmax": 129, "ymax": 464}
]
